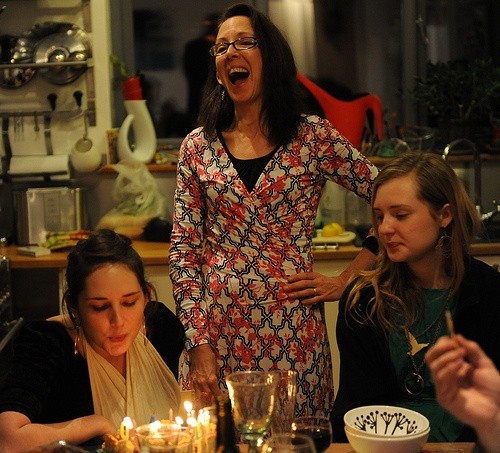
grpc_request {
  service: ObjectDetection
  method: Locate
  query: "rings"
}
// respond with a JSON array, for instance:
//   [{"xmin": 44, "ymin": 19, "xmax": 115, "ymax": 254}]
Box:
[{"xmin": 313, "ymin": 288, "xmax": 317, "ymax": 295}]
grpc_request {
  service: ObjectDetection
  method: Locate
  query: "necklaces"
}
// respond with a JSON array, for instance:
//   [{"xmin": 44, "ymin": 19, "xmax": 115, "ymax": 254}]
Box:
[{"xmin": 398, "ymin": 289, "xmax": 449, "ymax": 397}]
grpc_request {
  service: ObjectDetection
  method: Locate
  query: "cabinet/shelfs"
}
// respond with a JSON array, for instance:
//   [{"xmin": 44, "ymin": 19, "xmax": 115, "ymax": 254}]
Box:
[{"xmin": 0, "ymin": 0, "xmax": 97, "ymax": 125}]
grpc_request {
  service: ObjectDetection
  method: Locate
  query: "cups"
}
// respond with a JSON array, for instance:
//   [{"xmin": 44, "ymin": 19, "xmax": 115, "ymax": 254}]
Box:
[
  {"xmin": 261, "ymin": 369, "xmax": 332, "ymax": 453},
  {"xmin": 225, "ymin": 370, "xmax": 277, "ymax": 453}
]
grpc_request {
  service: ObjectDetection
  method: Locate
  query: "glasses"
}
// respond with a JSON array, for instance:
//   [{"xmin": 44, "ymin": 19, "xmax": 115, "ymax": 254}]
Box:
[{"xmin": 207, "ymin": 36, "xmax": 259, "ymax": 57}]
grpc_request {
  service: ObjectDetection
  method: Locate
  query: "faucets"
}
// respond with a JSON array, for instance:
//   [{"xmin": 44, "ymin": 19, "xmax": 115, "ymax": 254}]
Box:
[{"xmin": 442, "ymin": 138, "xmax": 482, "ymax": 212}]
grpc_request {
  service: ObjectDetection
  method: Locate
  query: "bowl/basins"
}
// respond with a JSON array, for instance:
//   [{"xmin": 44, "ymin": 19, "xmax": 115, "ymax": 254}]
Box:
[{"xmin": 343, "ymin": 405, "xmax": 431, "ymax": 453}]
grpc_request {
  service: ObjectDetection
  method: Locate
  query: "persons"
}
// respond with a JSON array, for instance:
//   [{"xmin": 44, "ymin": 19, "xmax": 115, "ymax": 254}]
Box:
[
  {"xmin": 168, "ymin": 4, "xmax": 382, "ymax": 443},
  {"xmin": 330, "ymin": 152, "xmax": 500, "ymax": 444},
  {"xmin": 184, "ymin": 14, "xmax": 221, "ymax": 134},
  {"xmin": 0, "ymin": 229, "xmax": 185, "ymax": 453},
  {"xmin": 425, "ymin": 335, "xmax": 500, "ymax": 453}
]
